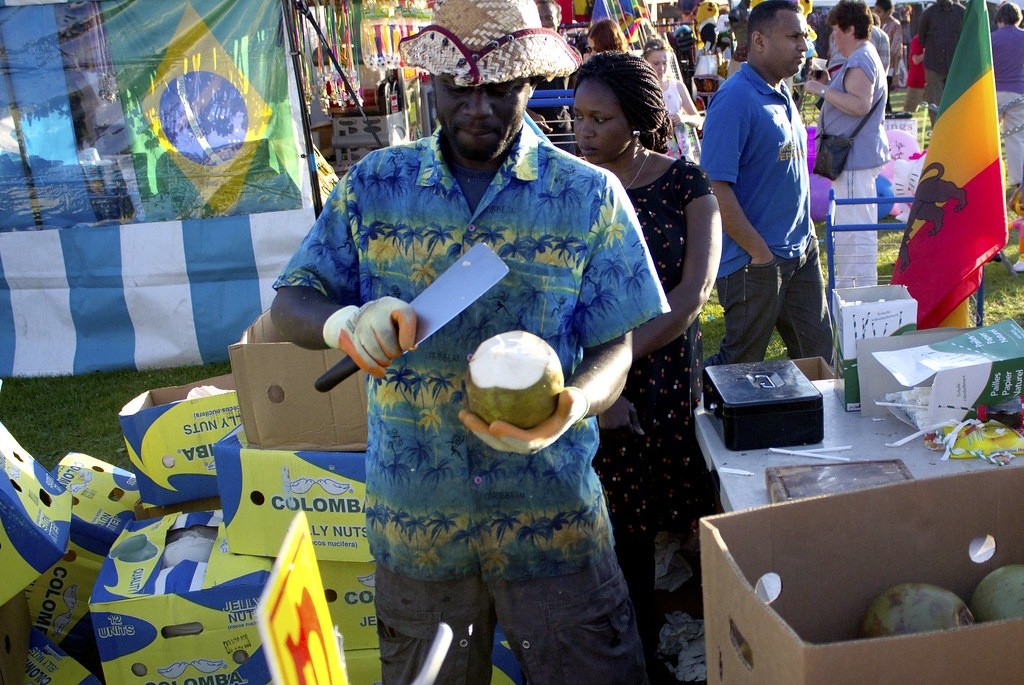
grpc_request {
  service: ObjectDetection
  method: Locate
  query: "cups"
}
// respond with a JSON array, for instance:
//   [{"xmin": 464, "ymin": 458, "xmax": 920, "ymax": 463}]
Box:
[{"xmin": 811, "ymin": 56, "xmax": 828, "ymax": 78}]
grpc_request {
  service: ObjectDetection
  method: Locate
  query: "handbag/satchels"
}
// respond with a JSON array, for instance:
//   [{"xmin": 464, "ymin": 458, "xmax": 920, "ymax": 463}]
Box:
[
  {"xmin": 813, "ymin": 131, "xmax": 858, "ymax": 181},
  {"xmin": 665, "ymin": 120, "xmax": 701, "ymax": 166},
  {"xmin": 892, "ymin": 61, "xmax": 908, "ymax": 89}
]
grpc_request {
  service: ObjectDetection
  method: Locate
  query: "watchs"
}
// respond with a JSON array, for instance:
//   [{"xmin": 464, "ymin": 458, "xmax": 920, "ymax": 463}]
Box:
[{"xmin": 819, "ymin": 85, "xmax": 826, "ymax": 97}]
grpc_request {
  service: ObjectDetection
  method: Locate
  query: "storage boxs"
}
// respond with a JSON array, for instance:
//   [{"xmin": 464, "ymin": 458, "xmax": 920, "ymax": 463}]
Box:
[
  {"xmin": 831, "ymin": 284, "xmax": 918, "ymax": 414},
  {"xmin": 871, "ymin": 319, "xmax": 1024, "ymax": 434},
  {"xmin": 791, "ymin": 355, "xmax": 836, "ymax": 380},
  {"xmin": 696, "ymin": 465, "xmax": 1024, "ymax": 685},
  {"xmin": 0, "ymin": 308, "xmax": 528, "ymax": 685},
  {"xmin": 332, "ymin": 110, "xmax": 409, "ymax": 166}
]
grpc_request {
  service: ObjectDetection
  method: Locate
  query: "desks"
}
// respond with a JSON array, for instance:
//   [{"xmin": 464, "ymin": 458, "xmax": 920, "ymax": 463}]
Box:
[{"xmin": 693, "ymin": 380, "xmax": 1024, "ymax": 515}]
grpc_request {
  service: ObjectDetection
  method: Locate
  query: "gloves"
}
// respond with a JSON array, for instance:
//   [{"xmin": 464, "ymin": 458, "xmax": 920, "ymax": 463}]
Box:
[
  {"xmin": 322, "ymin": 294, "xmax": 419, "ymax": 381},
  {"xmin": 458, "ymin": 385, "xmax": 591, "ymax": 456}
]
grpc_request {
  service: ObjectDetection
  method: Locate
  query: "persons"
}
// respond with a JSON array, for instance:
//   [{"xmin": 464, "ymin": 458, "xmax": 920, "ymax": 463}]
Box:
[
  {"xmin": 583, "ymin": 19, "xmax": 630, "ymax": 63},
  {"xmin": 640, "ymin": 39, "xmax": 703, "ymax": 171},
  {"xmin": 673, "ymin": 0, "xmax": 1024, "ymax": 129},
  {"xmin": 525, "ymin": 1, "xmax": 575, "ymax": 156},
  {"xmin": 990, "ymin": 3, "xmax": 1023, "ymax": 193},
  {"xmin": 270, "ymin": 0, "xmax": 671, "ymax": 685},
  {"xmin": 702, "ymin": 0, "xmax": 833, "ymax": 382},
  {"xmin": 1007, "ymin": 184, "xmax": 1023, "ymax": 272},
  {"xmin": 571, "ymin": 50, "xmax": 722, "ymax": 685},
  {"xmin": 802, "ymin": 0, "xmax": 891, "ymax": 291}
]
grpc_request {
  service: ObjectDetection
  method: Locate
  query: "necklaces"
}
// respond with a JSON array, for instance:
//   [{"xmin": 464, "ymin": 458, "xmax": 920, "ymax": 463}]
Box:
[{"xmin": 626, "ymin": 150, "xmax": 650, "ymax": 190}]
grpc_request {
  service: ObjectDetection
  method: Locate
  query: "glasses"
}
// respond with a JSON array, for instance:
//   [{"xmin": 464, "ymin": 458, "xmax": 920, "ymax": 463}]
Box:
[{"xmin": 585, "ymin": 45, "xmax": 601, "ymax": 53}]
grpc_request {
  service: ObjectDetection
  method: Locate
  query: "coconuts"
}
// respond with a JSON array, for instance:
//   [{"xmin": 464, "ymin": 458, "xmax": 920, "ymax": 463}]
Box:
[
  {"xmin": 466, "ymin": 329, "xmax": 566, "ymax": 430},
  {"xmin": 857, "ymin": 561, "xmax": 1024, "ymax": 638}
]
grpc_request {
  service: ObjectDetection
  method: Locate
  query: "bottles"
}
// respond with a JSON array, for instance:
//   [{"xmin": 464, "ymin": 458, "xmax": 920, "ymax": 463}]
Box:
[{"xmin": 978, "ymin": 396, "xmax": 1024, "ymax": 429}]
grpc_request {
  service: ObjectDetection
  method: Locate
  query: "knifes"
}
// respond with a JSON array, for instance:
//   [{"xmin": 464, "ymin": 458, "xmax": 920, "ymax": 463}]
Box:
[{"xmin": 316, "ymin": 243, "xmax": 509, "ymax": 393}]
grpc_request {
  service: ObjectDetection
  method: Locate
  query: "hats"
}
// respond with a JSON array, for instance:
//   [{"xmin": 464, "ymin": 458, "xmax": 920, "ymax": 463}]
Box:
[{"xmin": 384, "ymin": 0, "xmax": 586, "ymax": 88}]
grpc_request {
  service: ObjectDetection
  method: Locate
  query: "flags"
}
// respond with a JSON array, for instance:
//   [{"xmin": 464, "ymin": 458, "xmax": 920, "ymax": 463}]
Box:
[
  {"xmin": 889, "ymin": 0, "xmax": 1006, "ymax": 334},
  {"xmin": 589, "ymin": 0, "xmax": 653, "ymax": 51},
  {"xmin": 101, "ymin": 1, "xmax": 304, "ymax": 214}
]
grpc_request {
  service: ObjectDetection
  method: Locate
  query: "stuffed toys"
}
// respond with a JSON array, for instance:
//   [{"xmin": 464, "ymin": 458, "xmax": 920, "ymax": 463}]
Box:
[{"xmin": 800, "ymin": 130, "xmax": 941, "ymax": 222}]
